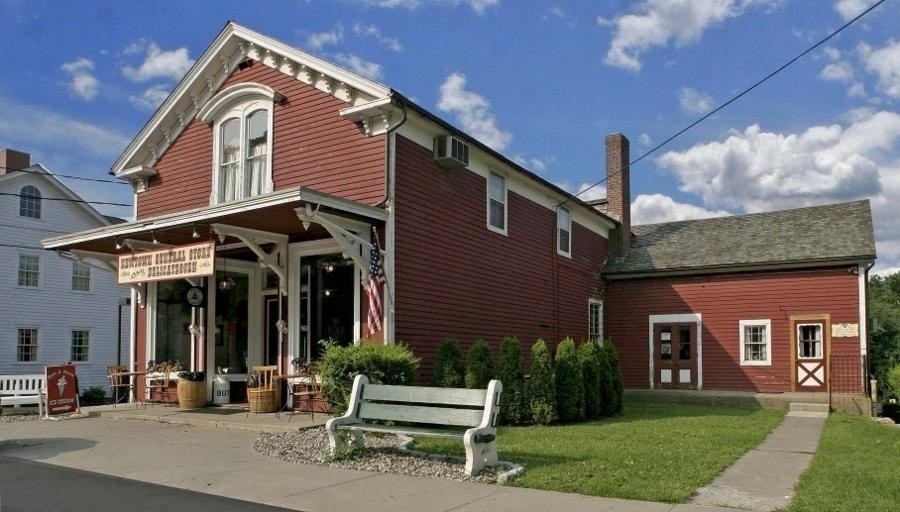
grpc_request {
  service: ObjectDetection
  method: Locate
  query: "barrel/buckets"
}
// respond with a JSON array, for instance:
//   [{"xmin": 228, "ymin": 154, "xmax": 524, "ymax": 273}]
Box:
[{"xmin": 177, "ymin": 371, "xmax": 207, "ymax": 410}]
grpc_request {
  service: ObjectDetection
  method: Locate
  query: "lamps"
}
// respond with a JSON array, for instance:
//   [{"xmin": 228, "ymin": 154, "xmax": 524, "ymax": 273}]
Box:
[
  {"xmin": 152, "ymin": 234, "xmax": 158, "ymax": 246},
  {"xmin": 325, "ymin": 288, "xmax": 330, "ymax": 296},
  {"xmin": 192, "ymin": 226, "xmax": 200, "ymax": 238},
  {"xmin": 218, "ymin": 239, "xmax": 234, "ymax": 290},
  {"xmin": 114, "ymin": 240, "xmax": 122, "ymax": 250}
]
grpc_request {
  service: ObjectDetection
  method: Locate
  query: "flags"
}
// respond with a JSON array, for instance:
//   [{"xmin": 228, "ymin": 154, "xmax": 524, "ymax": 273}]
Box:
[{"xmin": 366, "ymin": 235, "xmax": 387, "ymax": 339}]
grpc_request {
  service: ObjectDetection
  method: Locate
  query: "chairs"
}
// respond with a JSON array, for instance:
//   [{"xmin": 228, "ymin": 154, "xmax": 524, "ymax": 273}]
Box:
[
  {"xmin": 107, "ymin": 364, "xmax": 172, "ymax": 408},
  {"xmin": 245, "ymin": 365, "xmax": 322, "ymax": 422}
]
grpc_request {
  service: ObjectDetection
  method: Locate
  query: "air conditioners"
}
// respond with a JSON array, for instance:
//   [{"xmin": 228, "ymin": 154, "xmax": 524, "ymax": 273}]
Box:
[{"xmin": 434, "ymin": 133, "xmax": 469, "ymax": 169}]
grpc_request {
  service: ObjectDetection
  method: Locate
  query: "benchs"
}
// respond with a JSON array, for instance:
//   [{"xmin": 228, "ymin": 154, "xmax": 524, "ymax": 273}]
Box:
[
  {"xmin": 0, "ymin": 374, "xmax": 46, "ymax": 416},
  {"xmin": 325, "ymin": 375, "xmax": 504, "ymax": 477}
]
